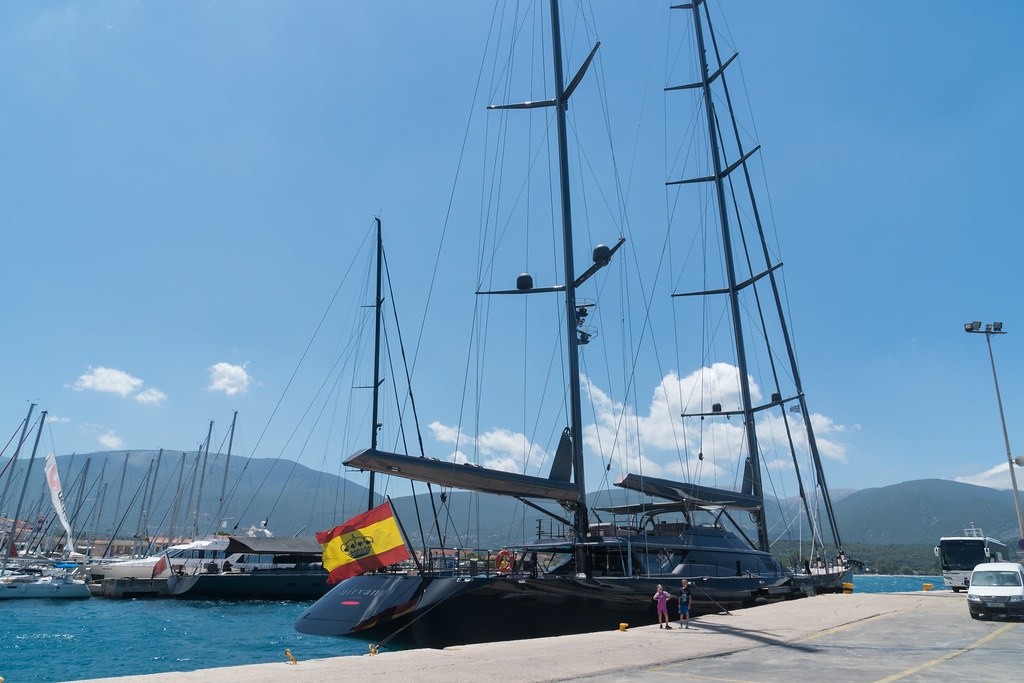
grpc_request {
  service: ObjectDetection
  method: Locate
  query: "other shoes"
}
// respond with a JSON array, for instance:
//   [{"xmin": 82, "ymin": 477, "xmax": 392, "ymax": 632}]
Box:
[
  {"xmin": 666, "ymin": 626, "xmax": 672, "ymax": 629},
  {"xmin": 660, "ymin": 626, "xmax": 662, "ymax": 628}
]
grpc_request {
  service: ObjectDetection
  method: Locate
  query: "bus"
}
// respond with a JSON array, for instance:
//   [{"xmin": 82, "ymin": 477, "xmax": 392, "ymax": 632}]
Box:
[{"xmin": 933, "ymin": 536, "xmax": 1009, "ymax": 593}]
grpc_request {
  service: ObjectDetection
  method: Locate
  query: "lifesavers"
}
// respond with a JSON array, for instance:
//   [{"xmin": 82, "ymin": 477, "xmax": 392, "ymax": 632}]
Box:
[{"xmin": 494, "ymin": 549, "xmax": 515, "ymax": 573}]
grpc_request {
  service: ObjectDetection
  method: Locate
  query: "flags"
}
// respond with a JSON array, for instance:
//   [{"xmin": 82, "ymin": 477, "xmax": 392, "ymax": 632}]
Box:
[
  {"xmin": 317, "ymin": 501, "xmax": 409, "ymax": 585},
  {"xmin": 148, "ymin": 555, "xmax": 169, "ymax": 588}
]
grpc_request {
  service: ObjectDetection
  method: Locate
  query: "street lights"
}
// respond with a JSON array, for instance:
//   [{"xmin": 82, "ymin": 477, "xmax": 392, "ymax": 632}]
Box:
[{"xmin": 963, "ymin": 321, "xmax": 1024, "ymax": 543}]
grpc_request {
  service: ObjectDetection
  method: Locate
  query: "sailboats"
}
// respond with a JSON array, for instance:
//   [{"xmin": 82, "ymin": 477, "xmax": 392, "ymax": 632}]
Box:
[
  {"xmin": 1, "ymin": 399, "xmax": 324, "ymax": 601},
  {"xmin": 165, "ymin": 217, "xmax": 500, "ymax": 603},
  {"xmin": 292, "ymin": 0, "xmax": 867, "ymax": 651}
]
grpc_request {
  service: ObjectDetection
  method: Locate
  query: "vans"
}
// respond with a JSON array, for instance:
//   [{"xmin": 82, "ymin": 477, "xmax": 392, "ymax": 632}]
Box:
[{"xmin": 963, "ymin": 562, "xmax": 1024, "ymax": 620}]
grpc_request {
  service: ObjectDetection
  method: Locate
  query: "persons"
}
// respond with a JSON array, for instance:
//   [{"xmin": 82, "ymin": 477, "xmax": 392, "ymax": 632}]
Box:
[
  {"xmin": 677, "ymin": 580, "xmax": 691, "ymax": 629},
  {"xmin": 653, "ymin": 584, "xmax": 673, "ymax": 629}
]
